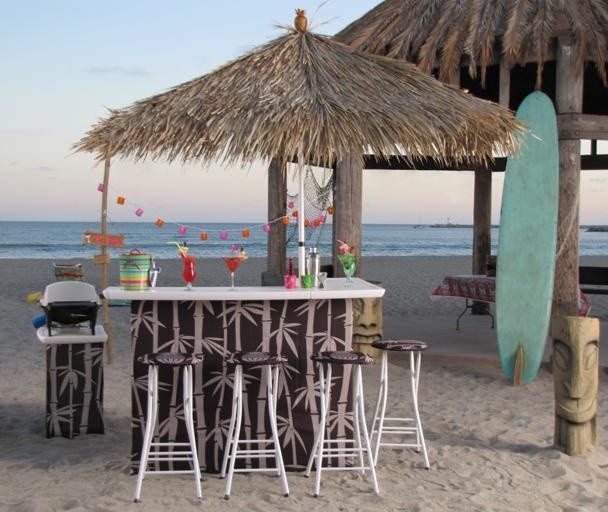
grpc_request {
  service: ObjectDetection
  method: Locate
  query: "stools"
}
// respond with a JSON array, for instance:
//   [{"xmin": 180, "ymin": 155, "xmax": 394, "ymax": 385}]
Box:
[
  {"xmin": 303, "ymin": 351, "xmax": 380, "ymax": 498},
  {"xmin": 219, "ymin": 352, "xmax": 290, "ymax": 500},
  {"xmin": 134, "ymin": 353, "xmax": 203, "ymax": 503},
  {"xmin": 368, "ymin": 340, "xmax": 430, "ymax": 470}
]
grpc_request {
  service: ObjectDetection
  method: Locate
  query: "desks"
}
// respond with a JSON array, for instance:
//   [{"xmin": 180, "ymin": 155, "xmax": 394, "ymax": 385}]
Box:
[{"xmin": 443, "ymin": 274, "xmax": 496, "ymax": 332}]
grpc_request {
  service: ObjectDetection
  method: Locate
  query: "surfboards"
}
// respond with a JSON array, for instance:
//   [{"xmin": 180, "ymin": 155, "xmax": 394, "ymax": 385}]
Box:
[{"xmin": 494, "ymin": 90, "xmax": 560, "ymax": 386}]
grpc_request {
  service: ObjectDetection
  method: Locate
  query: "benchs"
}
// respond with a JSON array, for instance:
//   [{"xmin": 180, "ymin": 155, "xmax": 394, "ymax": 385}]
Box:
[{"xmin": 578, "ymin": 265, "xmax": 608, "ymax": 296}]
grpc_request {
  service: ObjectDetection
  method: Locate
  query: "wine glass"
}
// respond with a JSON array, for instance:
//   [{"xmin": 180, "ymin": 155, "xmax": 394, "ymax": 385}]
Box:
[
  {"xmin": 337, "ymin": 253, "xmax": 360, "ymax": 286},
  {"xmin": 182, "ymin": 253, "xmax": 197, "ymax": 292},
  {"xmin": 221, "ymin": 254, "xmax": 243, "ymax": 292}
]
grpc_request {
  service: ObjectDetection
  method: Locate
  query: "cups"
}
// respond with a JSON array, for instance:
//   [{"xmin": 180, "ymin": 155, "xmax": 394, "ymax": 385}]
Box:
[{"xmin": 284, "ymin": 272, "xmax": 327, "ymax": 289}]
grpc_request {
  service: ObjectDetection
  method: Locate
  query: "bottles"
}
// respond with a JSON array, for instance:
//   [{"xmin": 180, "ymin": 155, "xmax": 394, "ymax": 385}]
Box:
[{"xmin": 306, "ymin": 247, "xmax": 321, "ymax": 289}]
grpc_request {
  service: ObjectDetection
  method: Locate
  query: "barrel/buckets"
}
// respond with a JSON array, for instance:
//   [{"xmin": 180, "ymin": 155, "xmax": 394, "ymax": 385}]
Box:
[
  {"xmin": 52, "ymin": 261, "xmax": 87, "ymax": 281},
  {"xmin": 115, "ymin": 248, "xmax": 155, "ymax": 291}
]
